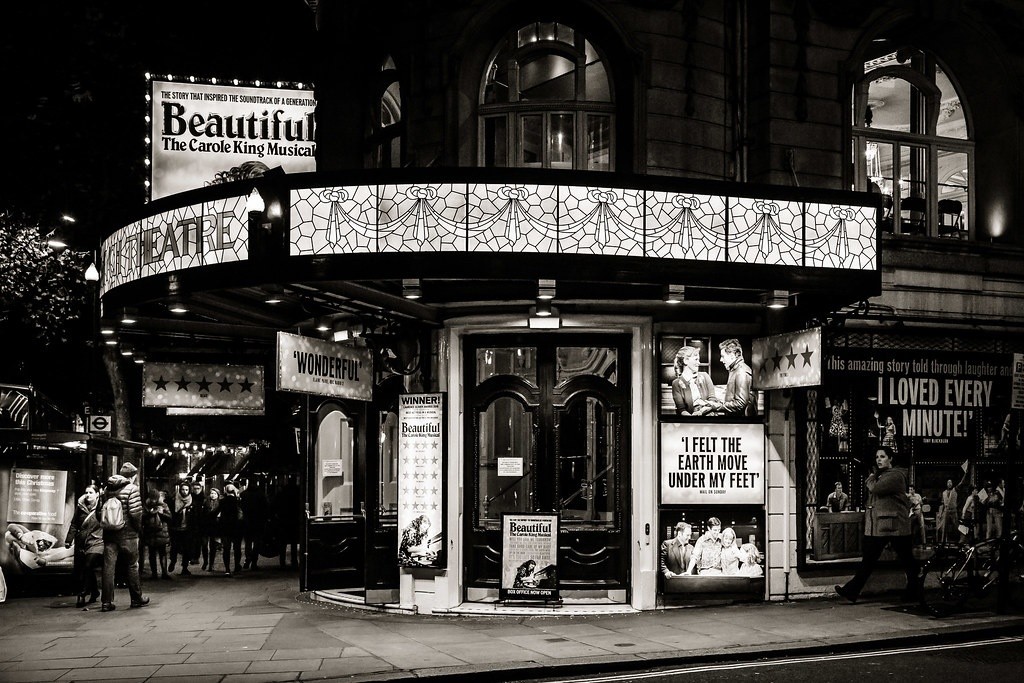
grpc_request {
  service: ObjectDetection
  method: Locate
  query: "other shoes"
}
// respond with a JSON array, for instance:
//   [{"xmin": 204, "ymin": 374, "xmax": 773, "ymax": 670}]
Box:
[
  {"xmin": 130, "ymin": 595, "xmax": 150, "ymax": 608},
  {"xmin": 89, "ymin": 591, "xmax": 100, "ymax": 603},
  {"xmin": 234, "ymin": 564, "xmax": 242, "ymax": 574},
  {"xmin": 202, "ymin": 563, "xmax": 209, "ymax": 570},
  {"xmin": 278, "ymin": 558, "xmax": 286, "ymax": 568},
  {"xmin": 243, "ymin": 561, "xmax": 259, "ymax": 571},
  {"xmin": 168, "ymin": 562, "xmax": 175, "ymax": 572},
  {"xmin": 189, "ymin": 558, "xmax": 200, "ymax": 565},
  {"xmin": 208, "ymin": 565, "xmax": 215, "ymax": 572},
  {"xmin": 834, "ymin": 584, "xmax": 857, "ymax": 603},
  {"xmin": 149, "ymin": 576, "xmax": 158, "ymax": 580},
  {"xmin": 101, "ymin": 603, "xmax": 115, "ymax": 612},
  {"xmin": 161, "ymin": 574, "xmax": 171, "ymax": 580},
  {"xmin": 291, "ymin": 559, "xmax": 299, "ymax": 568},
  {"xmin": 181, "ymin": 567, "xmax": 192, "ymax": 576},
  {"xmin": 224, "ymin": 568, "xmax": 231, "ymax": 576},
  {"xmin": 76, "ymin": 596, "xmax": 86, "ymax": 607}
]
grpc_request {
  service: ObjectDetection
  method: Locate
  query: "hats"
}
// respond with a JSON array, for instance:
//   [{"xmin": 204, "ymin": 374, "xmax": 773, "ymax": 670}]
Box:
[{"xmin": 120, "ymin": 462, "xmax": 138, "ymax": 476}]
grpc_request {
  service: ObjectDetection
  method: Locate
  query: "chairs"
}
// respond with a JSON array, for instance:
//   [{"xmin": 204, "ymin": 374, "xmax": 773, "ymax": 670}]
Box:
[{"xmin": 879, "ymin": 193, "xmax": 964, "ymax": 239}]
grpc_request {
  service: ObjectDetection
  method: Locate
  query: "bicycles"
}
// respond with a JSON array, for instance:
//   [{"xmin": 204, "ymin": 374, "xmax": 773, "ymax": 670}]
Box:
[{"xmin": 919, "ymin": 502, "xmax": 1024, "ymax": 617}]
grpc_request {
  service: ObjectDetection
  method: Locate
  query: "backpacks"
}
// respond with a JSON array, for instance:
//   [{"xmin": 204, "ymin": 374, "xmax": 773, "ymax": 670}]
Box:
[{"xmin": 101, "ymin": 483, "xmax": 132, "ymax": 530}]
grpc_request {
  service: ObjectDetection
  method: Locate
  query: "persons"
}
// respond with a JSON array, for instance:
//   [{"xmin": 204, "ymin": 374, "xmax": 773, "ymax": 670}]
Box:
[
  {"xmin": 660, "ymin": 518, "xmax": 764, "ymax": 579},
  {"xmin": 513, "ymin": 560, "xmax": 536, "ymax": 588},
  {"xmin": 101, "ymin": 462, "xmax": 150, "ymax": 611},
  {"xmin": 905, "ymin": 479, "xmax": 1005, "ymax": 544},
  {"xmin": 834, "ymin": 447, "xmax": 923, "ymax": 602},
  {"xmin": 882, "ymin": 417, "xmax": 899, "ymax": 453},
  {"xmin": 827, "ymin": 481, "xmax": 853, "ymax": 554},
  {"xmin": 999, "ymin": 414, "xmax": 1020, "ymax": 443},
  {"xmin": 65, "ymin": 485, "xmax": 104, "ymax": 608},
  {"xmin": 141, "ymin": 478, "xmax": 258, "ymax": 576},
  {"xmin": 672, "ymin": 339, "xmax": 753, "ymax": 416}
]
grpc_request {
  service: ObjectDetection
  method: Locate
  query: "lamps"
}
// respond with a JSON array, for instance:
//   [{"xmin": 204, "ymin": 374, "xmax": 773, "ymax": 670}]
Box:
[
  {"xmin": 537, "ymin": 280, "xmax": 557, "ymax": 298},
  {"xmin": 766, "ymin": 291, "xmax": 790, "ymax": 308},
  {"xmin": 663, "ymin": 285, "xmax": 686, "ymax": 303},
  {"xmin": 401, "ymin": 279, "xmax": 422, "ymax": 301},
  {"xmin": 84, "ymin": 263, "xmax": 101, "ymax": 294},
  {"xmin": 535, "ymin": 298, "xmax": 552, "ymax": 315},
  {"xmin": 247, "ymin": 187, "xmax": 272, "ymax": 232}
]
